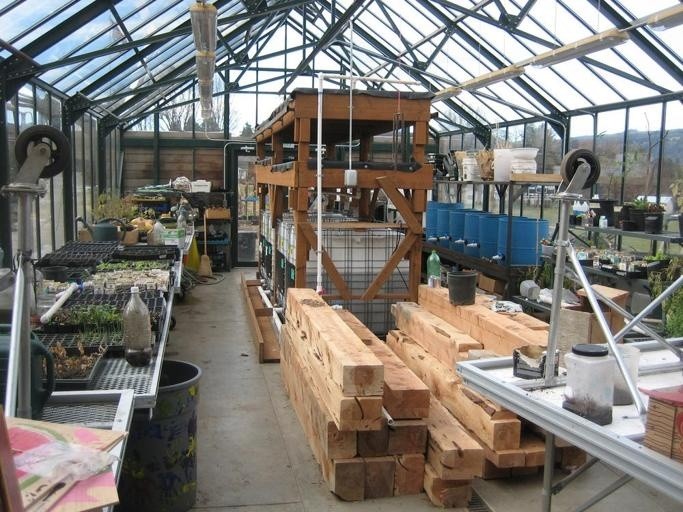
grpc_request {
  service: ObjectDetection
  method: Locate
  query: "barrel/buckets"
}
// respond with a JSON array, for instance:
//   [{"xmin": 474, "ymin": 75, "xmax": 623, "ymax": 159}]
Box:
[
  {"xmin": 572, "ymin": 198, "xmax": 591, "ymax": 227},
  {"xmin": 425, "ymin": 201, "xmax": 550, "ymax": 270},
  {"xmin": 448, "ymin": 269, "xmax": 480, "ymax": 306},
  {"xmin": 613, "ymin": 342, "xmax": 641, "ymax": 405},
  {"xmin": 493, "ymin": 149, "xmax": 514, "ymax": 182},
  {"xmin": 462, "ymin": 157, "xmax": 481, "ymax": 180}
]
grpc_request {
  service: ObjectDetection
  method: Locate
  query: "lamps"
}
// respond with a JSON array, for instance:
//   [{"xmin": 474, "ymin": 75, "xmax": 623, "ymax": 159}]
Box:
[
  {"xmin": 435, "ymin": 2, "xmax": 683, "ymax": 102},
  {"xmin": 189, "ymin": 2, "xmax": 216, "ymax": 122}
]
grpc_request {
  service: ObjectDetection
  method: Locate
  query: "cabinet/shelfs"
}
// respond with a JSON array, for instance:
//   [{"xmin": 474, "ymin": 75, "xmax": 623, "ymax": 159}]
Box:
[{"xmin": 119, "ymin": 183, "xmax": 233, "ymax": 271}]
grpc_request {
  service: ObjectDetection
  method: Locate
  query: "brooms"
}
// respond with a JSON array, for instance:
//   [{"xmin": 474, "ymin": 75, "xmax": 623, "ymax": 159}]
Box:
[{"xmin": 198, "ymin": 214, "xmax": 213, "ymax": 279}]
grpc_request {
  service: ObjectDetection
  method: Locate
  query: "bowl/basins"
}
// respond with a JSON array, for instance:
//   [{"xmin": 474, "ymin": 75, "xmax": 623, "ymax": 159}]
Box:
[{"xmin": 510, "ymin": 147, "xmax": 540, "ymax": 161}]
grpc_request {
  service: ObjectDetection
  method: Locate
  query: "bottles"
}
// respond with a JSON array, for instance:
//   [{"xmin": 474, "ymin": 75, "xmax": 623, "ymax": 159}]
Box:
[
  {"xmin": 153, "ymin": 218, "xmax": 167, "ymax": 246},
  {"xmin": 600, "ymin": 215, "xmax": 610, "ymax": 231},
  {"xmin": 561, "ymin": 342, "xmax": 617, "ymax": 427},
  {"xmin": 176, "ymin": 211, "xmax": 188, "ymax": 250},
  {"xmin": 425, "ymin": 248, "xmax": 442, "ymax": 285},
  {"xmin": 573, "ymin": 200, "xmax": 590, "ymax": 216},
  {"xmin": 121, "ymin": 285, "xmax": 154, "ymax": 368}
]
